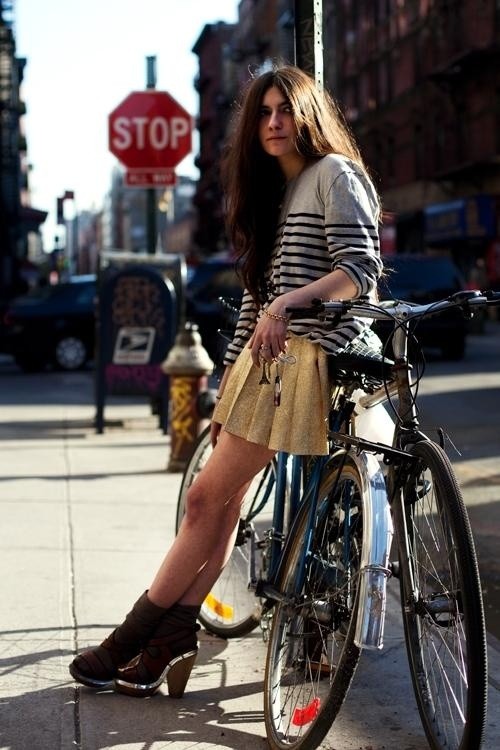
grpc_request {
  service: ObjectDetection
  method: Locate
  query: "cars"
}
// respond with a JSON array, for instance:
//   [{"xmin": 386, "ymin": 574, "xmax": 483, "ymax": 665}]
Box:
[
  {"xmin": 3, "ymin": 276, "xmax": 97, "ymax": 369},
  {"xmin": 381, "ymin": 253, "xmax": 485, "ymax": 361}
]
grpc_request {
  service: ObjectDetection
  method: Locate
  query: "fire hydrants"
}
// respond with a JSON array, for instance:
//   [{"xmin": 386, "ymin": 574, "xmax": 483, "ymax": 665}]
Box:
[{"xmin": 160, "ymin": 321, "xmax": 214, "ymax": 470}]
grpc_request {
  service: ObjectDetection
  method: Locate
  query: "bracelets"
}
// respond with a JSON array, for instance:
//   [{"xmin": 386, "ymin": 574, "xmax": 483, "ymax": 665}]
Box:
[
  {"xmin": 263, "ymin": 308, "xmax": 289, "ymax": 324},
  {"xmin": 215, "ymin": 395, "xmax": 221, "ymax": 399}
]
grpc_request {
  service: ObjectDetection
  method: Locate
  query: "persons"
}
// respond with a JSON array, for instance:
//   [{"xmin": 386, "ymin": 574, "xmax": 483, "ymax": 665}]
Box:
[{"xmin": 67, "ymin": 66, "xmax": 388, "ymax": 698}]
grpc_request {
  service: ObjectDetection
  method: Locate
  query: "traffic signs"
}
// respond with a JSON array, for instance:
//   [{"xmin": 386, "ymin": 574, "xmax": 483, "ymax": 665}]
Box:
[{"xmin": 125, "ymin": 169, "xmax": 176, "ymax": 186}]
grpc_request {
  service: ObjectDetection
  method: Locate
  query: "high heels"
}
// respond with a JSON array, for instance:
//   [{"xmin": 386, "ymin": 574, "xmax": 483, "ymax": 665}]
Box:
[
  {"xmin": 70, "ymin": 610, "xmax": 152, "ymax": 685},
  {"xmin": 116, "ymin": 621, "xmax": 200, "ymax": 698}
]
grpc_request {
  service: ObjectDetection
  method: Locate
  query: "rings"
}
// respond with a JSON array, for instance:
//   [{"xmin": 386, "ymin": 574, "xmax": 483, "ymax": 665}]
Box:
[{"xmin": 260, "ymin": 343, "xmax": 270, "ymax": 350}]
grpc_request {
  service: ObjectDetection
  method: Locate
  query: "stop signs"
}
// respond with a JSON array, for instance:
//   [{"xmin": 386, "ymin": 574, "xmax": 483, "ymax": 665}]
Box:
[{"xmin": 108, "ymin": 91, "xmax": 193, "ymax": 173}]
